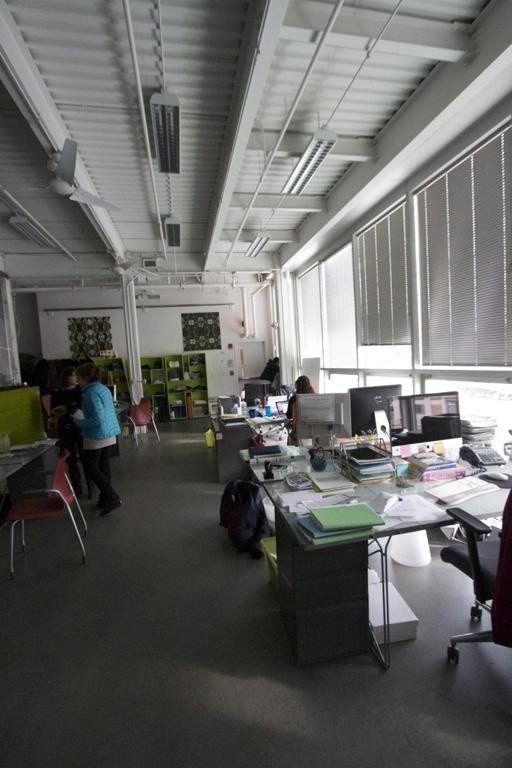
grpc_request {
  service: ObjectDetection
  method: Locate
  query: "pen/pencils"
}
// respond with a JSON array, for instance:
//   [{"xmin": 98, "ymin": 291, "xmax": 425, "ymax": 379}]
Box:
[{"xmin": 272, "ymin": 464, "xmax": 287, "ymax": 467}]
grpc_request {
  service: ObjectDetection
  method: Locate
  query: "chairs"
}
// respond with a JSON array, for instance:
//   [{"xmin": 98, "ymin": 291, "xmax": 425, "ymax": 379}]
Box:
[
  {"xmin": 6, "ymin": 449, "xmax": 89, "ymax": 579},
  {"xmin": 217, "ymin": 395, "xmax": 240, "ymax": 414},
  {"xmin": 121, "ymin": 397, "xmax": 152, "ymax": 442},
  {"xmin": 128, "ymin": 404, "xmax": 161, "ymax": 449}
]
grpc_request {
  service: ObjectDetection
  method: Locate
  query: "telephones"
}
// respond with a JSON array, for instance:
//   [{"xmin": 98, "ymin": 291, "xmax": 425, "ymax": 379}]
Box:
[{"xmin": 462, "ymin": 445, "xmax": 507, "ymax": 465}]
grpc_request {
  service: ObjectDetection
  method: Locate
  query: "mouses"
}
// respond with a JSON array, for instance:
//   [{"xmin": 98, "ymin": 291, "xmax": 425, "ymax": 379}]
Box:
[{"xmin": 486, "ymin": 471, "xmax": 510, "ymax": 481}]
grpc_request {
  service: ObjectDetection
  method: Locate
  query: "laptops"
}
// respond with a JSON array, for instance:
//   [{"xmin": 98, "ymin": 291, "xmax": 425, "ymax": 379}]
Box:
[{"xmin": 276, "ymin": 401, "xmax": 289, "ymax": 415}]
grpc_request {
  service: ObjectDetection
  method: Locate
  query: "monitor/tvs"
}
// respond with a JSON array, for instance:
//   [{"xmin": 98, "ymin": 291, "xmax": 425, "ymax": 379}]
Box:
[
  {"xmin": 245, "ymin": 383, "xmax": 266, "ymax": 402},
  {"xmin": 408, "ymin": 391, "xmax": 460, "ymax": 433},
  {"xmin": 348, "ymin": 384, "xmax": 402, "ymax": 445},
  {"xmin": 295, "ymin": 392, "xmax": 352, "ymax": 450}
]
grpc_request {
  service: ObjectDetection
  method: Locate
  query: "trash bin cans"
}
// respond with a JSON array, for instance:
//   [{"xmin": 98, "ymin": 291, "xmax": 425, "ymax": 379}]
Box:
[{"xmin": 205, "ymin": 428, "xmax": 216, "ymax": 447}]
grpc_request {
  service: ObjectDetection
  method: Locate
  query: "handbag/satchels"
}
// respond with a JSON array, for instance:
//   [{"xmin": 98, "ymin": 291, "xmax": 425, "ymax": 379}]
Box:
[{"xmin": 205, "ymin": 429, "xmax": 215, "ymax": 447}]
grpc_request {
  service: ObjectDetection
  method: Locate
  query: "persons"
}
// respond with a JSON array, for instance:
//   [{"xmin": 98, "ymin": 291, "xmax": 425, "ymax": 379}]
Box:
[
  {"xmin": 259, "ymin": 356, "xmax": 280, "ymax": 393},
  {"xmin": 285, "ymin": 375, "xmax": 316, "ymax": 445},
  {"xmin": 49, "ymin": 366, "xmax": 97, "ymax": 500},
  {"xmin": 67, "ymin": 362, "xmax": 123, "ymax": 516}
]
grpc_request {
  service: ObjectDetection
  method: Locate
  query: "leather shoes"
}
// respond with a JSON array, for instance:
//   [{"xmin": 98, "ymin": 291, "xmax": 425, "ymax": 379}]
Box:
[{"xmin": 97, "ymin": 497, "xmax": 123, "ymax": 516}]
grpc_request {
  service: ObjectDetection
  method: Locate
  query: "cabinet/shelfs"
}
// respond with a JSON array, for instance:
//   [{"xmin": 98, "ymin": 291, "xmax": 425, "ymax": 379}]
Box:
[{"xmin": 91, "ymin": 353, "xmax": 211, "ymax": 422}]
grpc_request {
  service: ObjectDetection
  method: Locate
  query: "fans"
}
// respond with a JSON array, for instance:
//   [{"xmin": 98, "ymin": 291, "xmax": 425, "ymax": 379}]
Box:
[
  {"xmin": 74, "ymin": 252, "xmax": 140, "ymax": 275},
  {"xmin": 22, "ymin": 138, "xmax": 125, "ymax": 215}
]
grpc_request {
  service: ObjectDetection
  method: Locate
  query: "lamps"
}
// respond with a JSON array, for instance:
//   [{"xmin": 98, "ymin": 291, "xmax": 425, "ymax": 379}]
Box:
[
  {"xmin": 244, "ymin": 232, "xmax": 270, "ymax": 257},
  {"xmin": 8, "ymin": 217, "xmax": 55, "ymax": 249},
  {"xmin": 279, "ymin": 129, "xmax": 337, "ymax": 195},
  {"xmin": 148, "ymin": 91, "xmax": 181, "ymax": 174},
  {"xmin": 163, "ymin": 217, "xmax": 180, "ymax": 248}
]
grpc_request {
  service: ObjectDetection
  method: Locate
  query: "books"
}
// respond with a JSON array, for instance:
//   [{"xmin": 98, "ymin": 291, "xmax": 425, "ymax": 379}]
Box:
[
  {"xmin": 239, "ymin": 412, "xmax": 512, "ymax": 546},
  {"xmin": 0, "ymin": 437, "xmax": 59, "ymax": 480}
]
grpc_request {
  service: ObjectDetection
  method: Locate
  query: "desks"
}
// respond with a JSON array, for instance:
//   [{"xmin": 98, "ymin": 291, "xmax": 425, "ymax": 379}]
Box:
[
  {"xmin": 115, "ymin": 401, "xmax": 128, "ymax": 416},
  {"xmin": 0, "ymin": 438, "xmax": 61, "ymax": 482},
  {"xmin": 208, "ymin": 415, "xmax": 294, "ymax": 482},
  {"xmin": 237, "ymin": 434, "xmax": 512, "ymax": 667}
]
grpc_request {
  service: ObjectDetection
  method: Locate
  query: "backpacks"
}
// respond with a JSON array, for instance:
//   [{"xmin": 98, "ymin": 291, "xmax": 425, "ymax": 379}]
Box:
[{"xmin": 219, "ymin": 480, "xmax": 270, "ymax": 559}]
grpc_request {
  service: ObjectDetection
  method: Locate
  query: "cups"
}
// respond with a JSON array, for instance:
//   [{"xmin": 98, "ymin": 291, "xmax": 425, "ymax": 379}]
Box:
[{"xmin": 265, "ymin": 405, "xmax": 271, "ymax": 417}]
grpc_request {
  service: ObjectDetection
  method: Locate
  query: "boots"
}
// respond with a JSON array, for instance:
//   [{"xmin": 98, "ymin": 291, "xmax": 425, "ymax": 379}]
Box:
[
  {"xmin": 85, "ymin": 474, "xmax": 96, "ymax": 498},
  {"xmin": 70, "ymin": 472, "xmax": 81, "ymax": 495}
]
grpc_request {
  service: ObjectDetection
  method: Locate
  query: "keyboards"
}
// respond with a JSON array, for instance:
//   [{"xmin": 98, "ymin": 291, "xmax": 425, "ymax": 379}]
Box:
[{"xmin": 425, "ymin": 476, "xmax": 500, "ymax": 505}]
